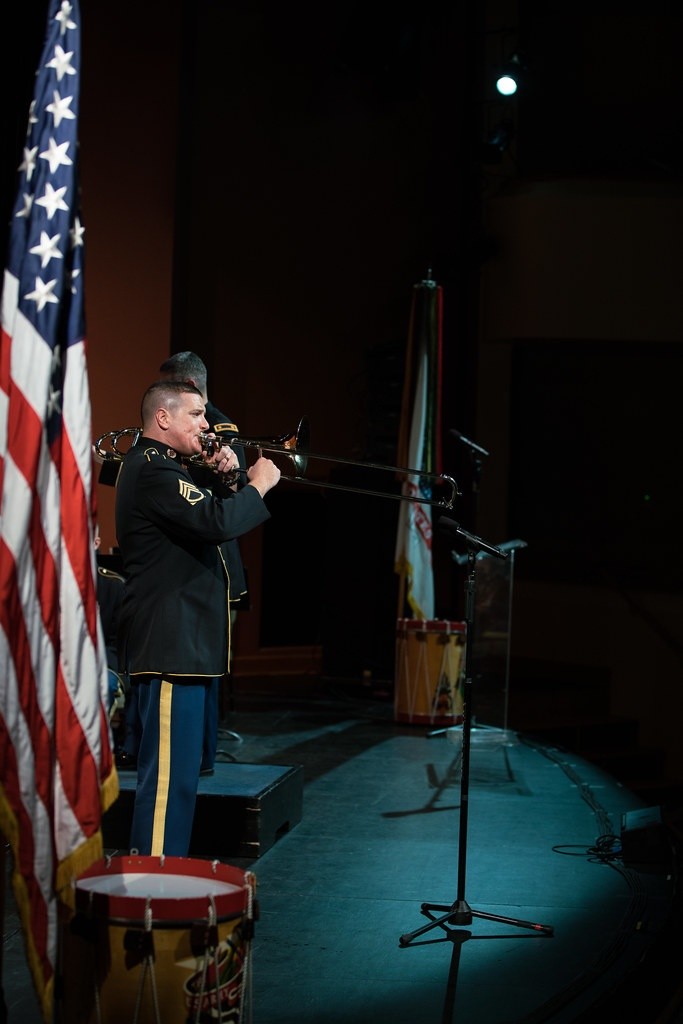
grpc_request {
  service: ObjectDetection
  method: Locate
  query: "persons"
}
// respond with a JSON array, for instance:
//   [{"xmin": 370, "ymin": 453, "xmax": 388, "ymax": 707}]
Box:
[
  {"xmin": 114, "ymin": 381, "xmax": 281, "ymax": 858},
  {"xmin": 93, "ymin": 351, "xmax": 249, "ymax": 775}
]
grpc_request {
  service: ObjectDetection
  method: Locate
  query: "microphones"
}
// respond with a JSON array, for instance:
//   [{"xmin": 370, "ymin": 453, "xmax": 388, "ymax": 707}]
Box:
[
  {"xmin": 438, "ymin": 515, "xmax": 509, "ymax": 560},
  {"xmin": 447, "ymin": 430, "xmax": 489, "ymax": 456}
]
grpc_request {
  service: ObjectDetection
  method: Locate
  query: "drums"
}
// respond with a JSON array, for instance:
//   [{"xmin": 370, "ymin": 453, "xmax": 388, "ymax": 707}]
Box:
[
  {"xmin": 54, "ymin": 854, "xmax": 258, "ymax": 1024},
  {"xmin": 393, "ymin": 617, "xmax": 468, "ymax": 726}
]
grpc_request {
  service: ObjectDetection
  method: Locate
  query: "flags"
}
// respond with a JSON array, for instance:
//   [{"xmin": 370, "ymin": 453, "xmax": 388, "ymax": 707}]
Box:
[
  {"xmin": 395, "ymin": 284, "xmax": 445, "ymax": 621},
  {"xmin": 0, "ymin": 3, "xmax": 120, "ymax": 1024}
]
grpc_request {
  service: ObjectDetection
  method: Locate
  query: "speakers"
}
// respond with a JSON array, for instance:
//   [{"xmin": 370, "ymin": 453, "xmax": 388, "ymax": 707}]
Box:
[{"xmin": 618, "ymin": 805, "xmax": 671, "ymax": 850}]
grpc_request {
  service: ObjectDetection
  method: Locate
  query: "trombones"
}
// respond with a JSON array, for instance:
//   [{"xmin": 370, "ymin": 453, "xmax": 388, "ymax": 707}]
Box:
[{"xmin": 93, "ymin": 415, "xmax": 459, "ymax": 511}]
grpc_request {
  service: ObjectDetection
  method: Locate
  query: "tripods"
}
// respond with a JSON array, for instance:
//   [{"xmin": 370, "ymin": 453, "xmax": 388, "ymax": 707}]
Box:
[{"xmin": 398, "ymin": 450, "xmax": 555, "ymax": 945}]
